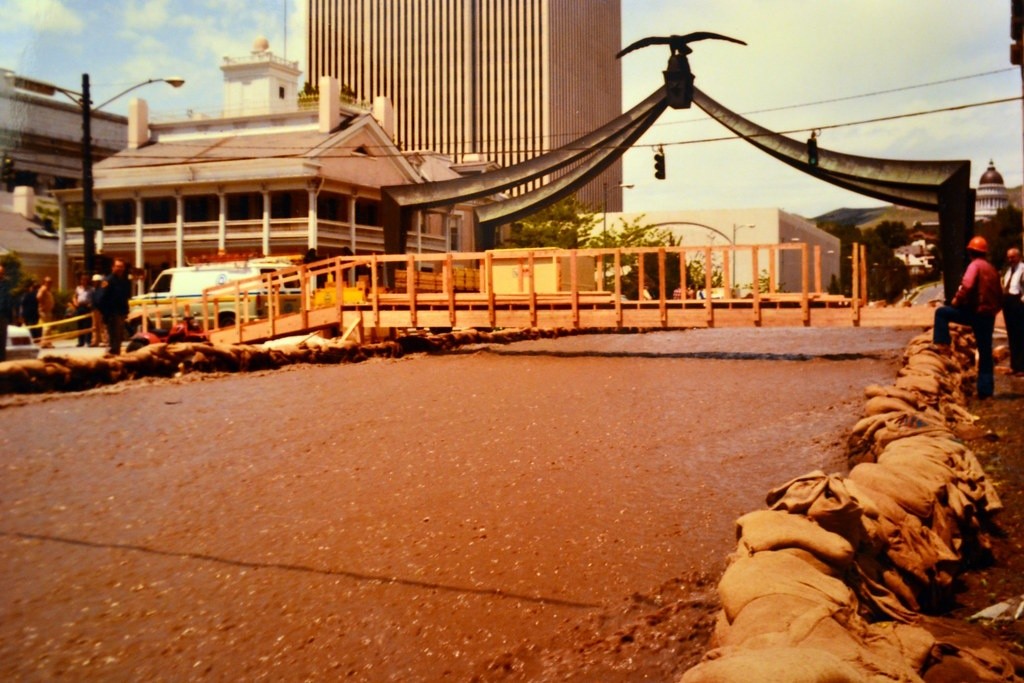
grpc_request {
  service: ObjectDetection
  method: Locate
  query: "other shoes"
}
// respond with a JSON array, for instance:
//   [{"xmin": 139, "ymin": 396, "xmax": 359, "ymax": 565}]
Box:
[
  {"xmin": 927, "ymin": 342, "xmax": 951, "ymax": 354},
  {"xmin": 1003, "ymin": 369, "xmax": 1024, "ymax": 379}
]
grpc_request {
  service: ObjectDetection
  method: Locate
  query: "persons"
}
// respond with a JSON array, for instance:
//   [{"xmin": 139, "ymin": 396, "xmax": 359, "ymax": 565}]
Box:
[
  {"xmin": 304, "ymin": 248, "xmax": 322, "ymax": 289},
  {"xmin": 0, "ymin": 264, "xmax": 55, "ymax": 348},
  {"xmin": 73, "ymin": 260, "xmax": 130, "ymax": 355},
  {"xmin": 673, "ymin": 283, "xmax": 704, "ymax": 300},
  {"xmin": 1001, "ymin": 249, "xmax": 1024, "ymax": 376},
  {"xmin": 630, "ymin": 266, "xmax": 649, "ymax": 299},
  {"xmin": 924, "ymin": 236, "xmax": 1002, "ymax": 399}
]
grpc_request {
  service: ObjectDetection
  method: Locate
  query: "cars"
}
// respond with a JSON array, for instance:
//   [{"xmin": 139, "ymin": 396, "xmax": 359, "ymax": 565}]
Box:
[{"xmin": 5, "ymin": 325, "xmax": 40, "ymax": 359}]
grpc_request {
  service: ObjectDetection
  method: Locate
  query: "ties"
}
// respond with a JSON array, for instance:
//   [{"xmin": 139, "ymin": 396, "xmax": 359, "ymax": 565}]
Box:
[{"xmin": 1004, "ymin": 266, "xmax": 1016, "ymax": 294}]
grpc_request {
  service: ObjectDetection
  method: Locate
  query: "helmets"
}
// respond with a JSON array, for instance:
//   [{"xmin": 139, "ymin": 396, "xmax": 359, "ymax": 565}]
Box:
[
  {"xmin": 92, "ymin": 274, "xmax": 102, "ymax": 282},
  {"xmin": 967, "ymin": 235, "xmax": 988, "ymax": 252}
]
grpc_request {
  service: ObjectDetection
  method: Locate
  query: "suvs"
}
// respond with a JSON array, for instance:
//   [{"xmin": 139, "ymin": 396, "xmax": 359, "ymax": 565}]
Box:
[{"xmin": 130, "ymin": 256, "xmax": 311, "ymax": 327}]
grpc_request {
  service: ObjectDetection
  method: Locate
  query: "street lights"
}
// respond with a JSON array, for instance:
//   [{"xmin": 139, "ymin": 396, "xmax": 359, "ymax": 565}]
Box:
[
  {"xmin": 602, "ymin": 182, "xmax": 634, "ymax": 291},
  {"xmin": 733, "ymin": 222, "xmax": 755, "ymax": 296},
  {"xmin": 82, "ymin": 74, "xmax": 185, "ymax": 284},
  {"xmin": 777, "ymin": 237, "xmax": 799, "ymax": 309},
  {"xmin": 826, "ymin": 251, "xmax": 834, "ymax": 307}
]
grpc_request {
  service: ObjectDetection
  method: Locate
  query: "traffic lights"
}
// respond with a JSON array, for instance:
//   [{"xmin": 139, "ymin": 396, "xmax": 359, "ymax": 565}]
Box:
[
  {"xmin": 807, "ymin": 139, "xmax": 818, "ymax": 167},
  {"xmin": 654, "ymin": 154, "xmax": 666, "ymax": 179}
]
